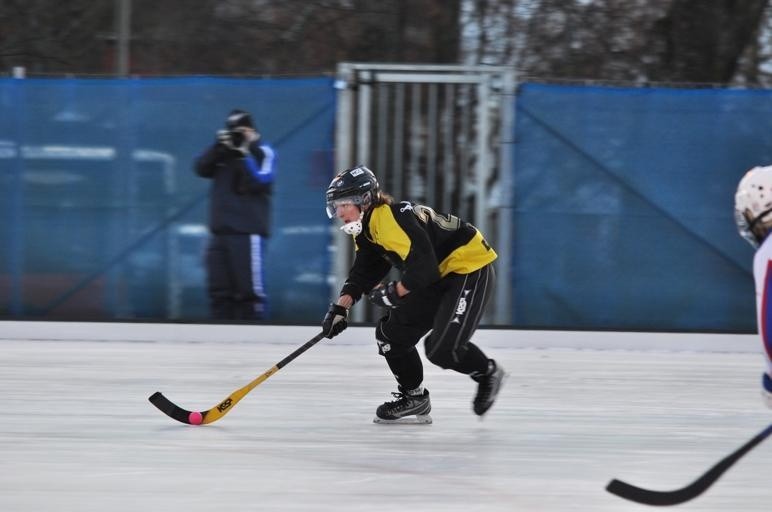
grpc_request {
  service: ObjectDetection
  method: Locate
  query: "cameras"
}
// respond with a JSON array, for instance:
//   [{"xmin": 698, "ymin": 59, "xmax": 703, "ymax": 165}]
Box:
[{"xmin": 230, "ymin": 130, "xmax": 244, "ymax": 148}]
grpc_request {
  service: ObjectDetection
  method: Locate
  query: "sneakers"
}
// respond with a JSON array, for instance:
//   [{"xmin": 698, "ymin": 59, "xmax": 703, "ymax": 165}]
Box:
[
  {"xmin": 210, "ymin": 304, "xmax": 271, "ymax": 325},
  {"xmin": 470, "ymin": 357, "xmax": 506, "ymax": 414},
  {"xmin": 376, "ymin": 387, "xmax": 432, "ymax": 420}
]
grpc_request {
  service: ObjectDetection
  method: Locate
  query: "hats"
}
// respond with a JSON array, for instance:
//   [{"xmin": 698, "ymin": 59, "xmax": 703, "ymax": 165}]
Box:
[{"xmin": 225, "ymin": 110, "xmax": 252, "ymax": 130}]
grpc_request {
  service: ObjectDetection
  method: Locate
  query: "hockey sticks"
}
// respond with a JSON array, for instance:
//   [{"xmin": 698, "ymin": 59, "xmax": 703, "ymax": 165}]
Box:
[
  {"xmin": 606, "ymin": 425, "xmax": 772, "ymax": 506},
  {"xmin": 147, "ymin": 324, "xmax": 350, "ymax": 424}
]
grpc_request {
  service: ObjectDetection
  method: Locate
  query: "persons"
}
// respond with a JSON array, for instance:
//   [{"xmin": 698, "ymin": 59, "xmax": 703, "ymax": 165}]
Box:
[
  {"xmin": 314, "ymin": 161, "xmax": 509, "ymax": 423},
  {"xmin": 731, "ymin": 164, "xmax": 772, "ymax": 420},
  {"xmin": 189, "ymin": 106, "xmax": 282, "ymax": 325}
]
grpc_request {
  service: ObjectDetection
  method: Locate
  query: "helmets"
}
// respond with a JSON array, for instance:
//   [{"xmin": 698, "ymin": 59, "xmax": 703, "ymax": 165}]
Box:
[
  {"xmin": 734, "ymin": 164, "xmax": 772, "ymax": 225},
  {"xmin": 326, "ymin": 165, "xmax": 380, "ymax": 201}
]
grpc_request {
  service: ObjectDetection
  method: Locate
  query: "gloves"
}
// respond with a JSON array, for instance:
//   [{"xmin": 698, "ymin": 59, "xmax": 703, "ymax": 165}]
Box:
[
  {"xmin": 368, "ymin": 280, "xmax": 403, "ymax": 311},
  {"xmin": 322, "ymin": 302, "xmax": 349, "ymax": 339},
  {"xmin": 214, "ymin": 130, "xmax": 249, "ymax": 159}
]
